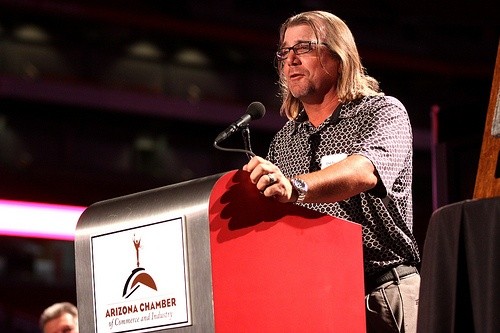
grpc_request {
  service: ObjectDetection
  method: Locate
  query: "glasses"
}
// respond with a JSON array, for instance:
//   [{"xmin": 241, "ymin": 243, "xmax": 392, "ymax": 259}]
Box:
[{"xmin": 274, "ymin": 40, "xmax": 329, "ymax": 61}]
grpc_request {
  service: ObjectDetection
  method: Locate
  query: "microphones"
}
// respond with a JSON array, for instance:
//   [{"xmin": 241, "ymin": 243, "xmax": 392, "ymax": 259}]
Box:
[{"xmin": 215, "ymin": 101, "xmax": 266, "ymax": 143}]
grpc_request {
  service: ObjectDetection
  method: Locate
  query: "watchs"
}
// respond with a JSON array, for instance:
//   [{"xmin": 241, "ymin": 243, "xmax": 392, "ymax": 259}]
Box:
[{"xmin": 289, "ymin": 175, "xmax": 307, "ymax": 205}]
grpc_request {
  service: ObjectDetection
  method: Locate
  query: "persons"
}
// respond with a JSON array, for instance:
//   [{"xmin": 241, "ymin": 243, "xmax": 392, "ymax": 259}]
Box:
[
  {"xmin": 242, "ymin": 11, "xmax": 422, "ymax": 333},
  {"xmin": 39, "ymin": 302, "xmax": 79, "ymax": 333},
  {"xmin": 416, "ymin": 195, "xmax": 500, "ymax": 333}
]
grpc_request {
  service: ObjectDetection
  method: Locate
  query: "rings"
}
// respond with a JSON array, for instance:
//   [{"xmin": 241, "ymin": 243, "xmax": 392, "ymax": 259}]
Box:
[{"xmin": 268, "ymin": 173, "xmax": 275, "ymax": 184}]
